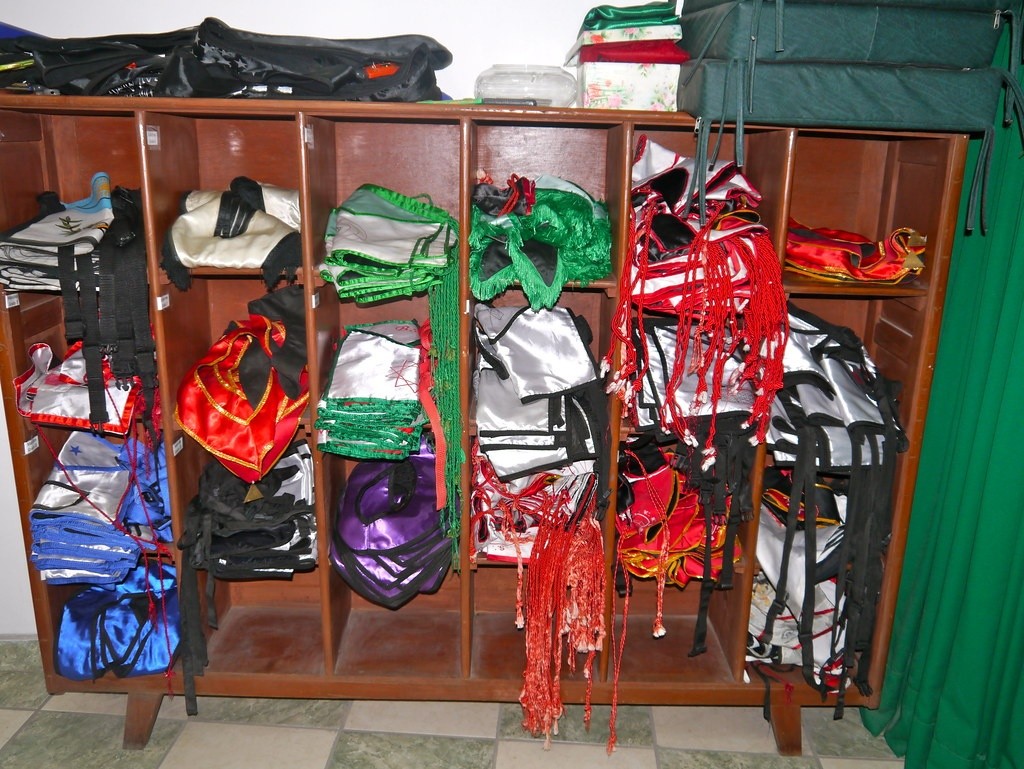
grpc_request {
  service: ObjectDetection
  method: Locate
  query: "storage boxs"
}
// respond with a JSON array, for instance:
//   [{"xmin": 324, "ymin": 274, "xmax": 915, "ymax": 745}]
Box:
[{"xmin": 564, "ymin": 25, "xmax": 691, "ymax": 112}]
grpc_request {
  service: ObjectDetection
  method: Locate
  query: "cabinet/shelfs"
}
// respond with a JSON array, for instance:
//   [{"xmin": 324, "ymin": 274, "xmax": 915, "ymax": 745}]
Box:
[{"xmin": 0, "ymin": 94, "xmax": 969, "ymax": 759}]
